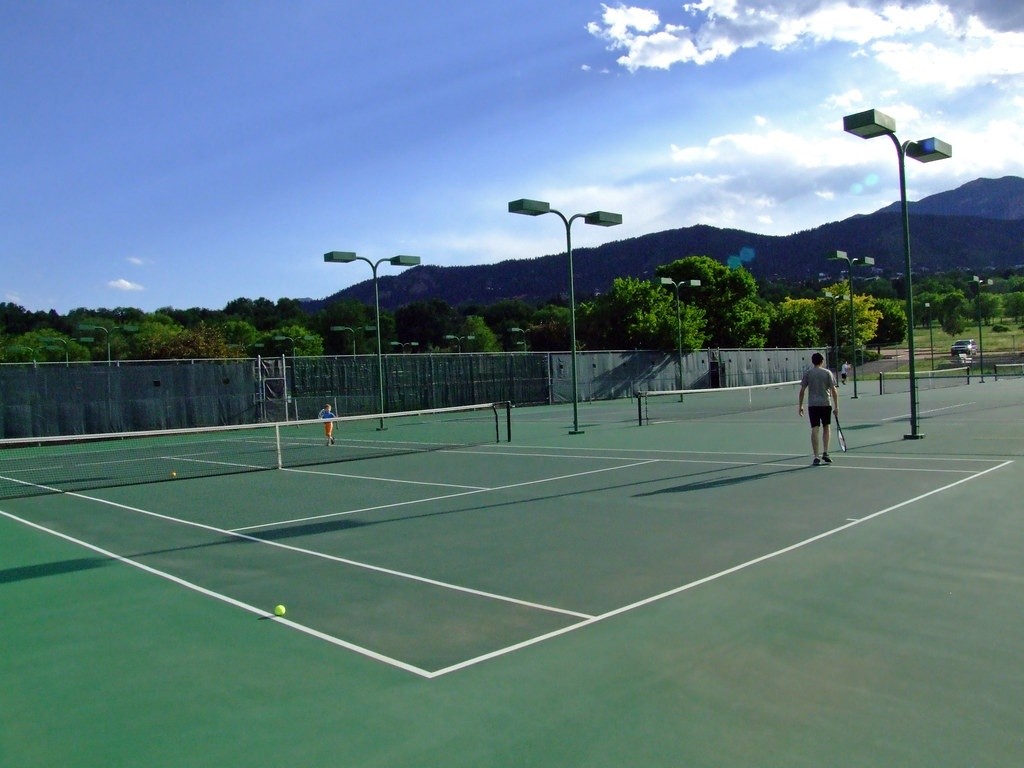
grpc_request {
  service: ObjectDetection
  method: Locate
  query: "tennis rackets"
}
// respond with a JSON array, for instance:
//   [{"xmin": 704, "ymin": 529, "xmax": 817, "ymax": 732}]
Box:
[
  {"xmin": 835, "ymin": 410, "xmax": 847, "ymax": 452},
  {"xmin": 318, "ymin": 408, "xmax": 325, "ymax": 418}
]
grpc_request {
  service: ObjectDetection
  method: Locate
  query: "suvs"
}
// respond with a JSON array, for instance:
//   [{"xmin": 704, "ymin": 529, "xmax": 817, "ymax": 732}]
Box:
[{"xmin": 951, "ymin": 339, "xmax": 977, "ymax": 355}]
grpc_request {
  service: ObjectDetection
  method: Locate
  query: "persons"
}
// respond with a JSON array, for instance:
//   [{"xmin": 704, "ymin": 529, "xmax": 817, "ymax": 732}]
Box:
[
  {"xmin": 841, "ymin": 360, "xmax": 851, "ymax": 385},
  {"xmin": 320, "ymin": 403, "xmax": 339, "ymax": 446},
  {"xmin": 798, "ymin": 353, "xmax": 839, "ymax": 465}
]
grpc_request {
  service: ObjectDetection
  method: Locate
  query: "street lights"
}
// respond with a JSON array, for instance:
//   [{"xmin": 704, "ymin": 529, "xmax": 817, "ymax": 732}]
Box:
[
  {"xmin": 508, "ymin": 199, "xmax": 622, "ymax": 435},
  {"xmin": 658, "ymin": 277, "xmax": 701, "ymax": 403},
  {"xmin": 843, "ymin": 108, "xmax": 952, "ymax": 440},
  {"xmin": 323, "ymin": 252, "xmax": 420, "ymax": 431},
  {"xmin": 825, "ymin": 250, "xmax": 875, "ymax": 399}
]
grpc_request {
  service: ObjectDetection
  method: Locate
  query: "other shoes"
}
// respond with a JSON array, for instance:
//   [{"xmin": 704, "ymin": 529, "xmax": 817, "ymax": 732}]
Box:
[
  {"xmin": 822, "ymin": 452, "xmax": 833, "ymax": 463},
  {"xmin": 813, "ymin": 458, "xmax": 821, "ymax": 466},
  {"xmin": 327, "ymin": 443, "xmax": 332, "ymax": 446},
  {"xmin": 331, "ymin": 437, "xmax": 335, "ymax": 444}
]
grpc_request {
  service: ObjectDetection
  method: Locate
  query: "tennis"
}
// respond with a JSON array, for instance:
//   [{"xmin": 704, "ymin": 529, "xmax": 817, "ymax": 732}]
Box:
[
  {"xmin": 275, "ymin": 604, "xmax": 287, "ymax": 616},
  {"xmin": 172, "ymin": 472, "xmax": 177, "ymax": 477}
]
